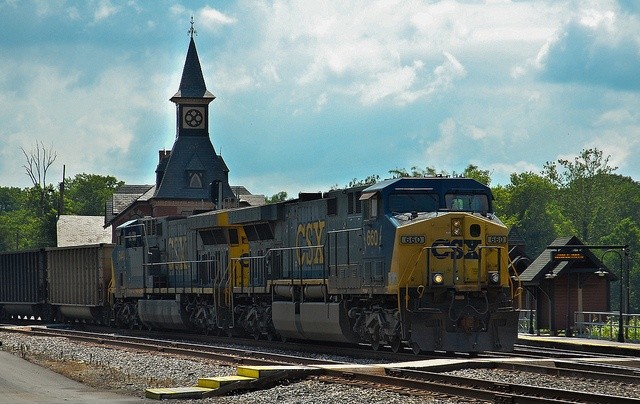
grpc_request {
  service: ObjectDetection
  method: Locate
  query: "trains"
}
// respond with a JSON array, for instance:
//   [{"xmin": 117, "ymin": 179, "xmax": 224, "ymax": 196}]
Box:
[{"xmin": 0, "ymin": 174, "xmax": 523, "ymax": 357}]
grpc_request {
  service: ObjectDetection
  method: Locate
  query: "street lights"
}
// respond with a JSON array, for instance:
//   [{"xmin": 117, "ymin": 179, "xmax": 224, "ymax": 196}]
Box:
[{"xmin": 594, "ymin": 249, "xmax": 624, "ymax": 342}]
